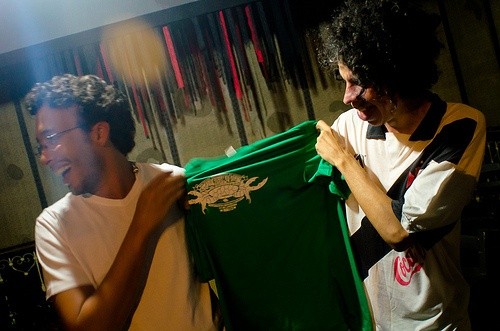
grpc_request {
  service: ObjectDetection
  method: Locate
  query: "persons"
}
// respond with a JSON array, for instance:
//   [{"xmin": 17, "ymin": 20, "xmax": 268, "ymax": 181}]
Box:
[
  {"xmin": 315, "ymin": 0, "xmax": 488, "ymax": 331},
  {"xmin": 26, "ymin": 74, "xmax": 215, "ymax": 331}
]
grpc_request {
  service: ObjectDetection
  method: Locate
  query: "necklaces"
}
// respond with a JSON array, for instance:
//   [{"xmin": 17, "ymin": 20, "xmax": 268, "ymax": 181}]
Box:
[{"xmin": 129, "ymin": 160, "xmax": 139, "ymax": 178}]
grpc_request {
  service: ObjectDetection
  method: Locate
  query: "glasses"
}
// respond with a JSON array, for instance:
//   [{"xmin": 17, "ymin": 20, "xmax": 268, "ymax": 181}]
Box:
[{"xmin": 33, "ymin": 122, "xmax": 89, "ymax": 159}]
